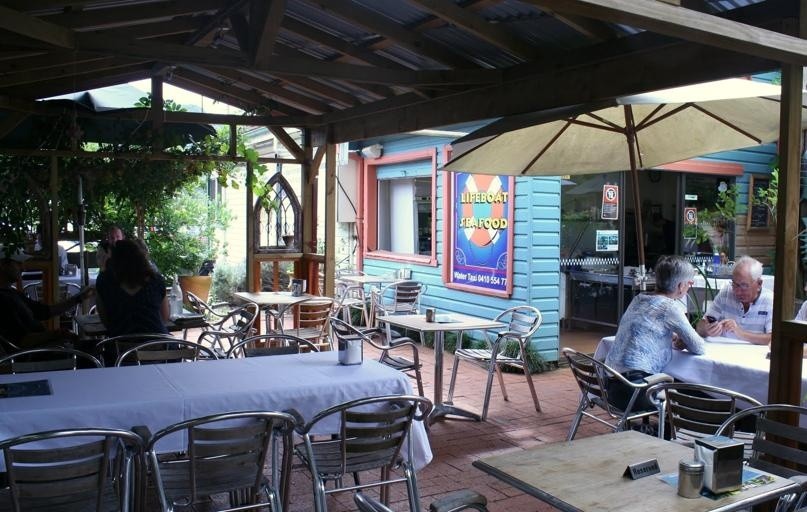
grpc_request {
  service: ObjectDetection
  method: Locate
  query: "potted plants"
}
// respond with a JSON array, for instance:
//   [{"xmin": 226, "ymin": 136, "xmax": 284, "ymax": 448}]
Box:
[{"xmin": 178, "ymin": 235, "xmax": 228, "ymax": 309}]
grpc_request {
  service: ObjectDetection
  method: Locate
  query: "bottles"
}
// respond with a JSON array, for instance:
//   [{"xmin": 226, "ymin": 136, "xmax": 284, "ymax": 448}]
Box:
[
  {"xmin": 713, "ymin": 253, "xmax": 721, "ymax": 275},
  {"xmin": 291, "ymin": 278, "xmax": 302, "ymax": 297},
  {"xmin": 425, "ymin": 307, "xmax": 435, "ymax": 322},
  {"xmin": 169, "ymin": 274, "xmax": 184, "ymax": 321},
  {"xmin": 678, "ymin": 458, "xmax": 705, "ymax": 499}
]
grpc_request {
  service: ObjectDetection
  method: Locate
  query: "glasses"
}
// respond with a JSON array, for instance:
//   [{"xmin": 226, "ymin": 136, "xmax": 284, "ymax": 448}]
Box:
[
  {"xmin": 688, "ymin": 280, "xmax": 694, "ymax": 285},
  {"xmin": 731, "ymin": 279, "xmax": 755, "ymax": 289}
]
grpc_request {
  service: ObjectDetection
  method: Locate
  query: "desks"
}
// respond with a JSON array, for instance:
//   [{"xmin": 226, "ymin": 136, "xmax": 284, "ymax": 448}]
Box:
[
  {"xmin": 376, "ymin": 311, "xmax": 508, "ymax": 427},
  {"xmin": 688, "ymin": 274, "xmax": 774, "ymax": 313},
  {"xmin": 570, "ymin": 266, "xmax": 657, "ymax": 290},
  {"xmin": 472, "ymin": 429, "xmax": 803, "ymax": 511},
  {"xmin": 580, "ymin": 335, "xmax": 807, "ymax": 429}
]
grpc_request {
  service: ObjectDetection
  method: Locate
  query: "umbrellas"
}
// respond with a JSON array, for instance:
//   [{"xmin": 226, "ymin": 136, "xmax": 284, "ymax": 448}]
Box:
[
  {"xmin": 34, "ymin": 84, "xmax": 221, "ymax": 319},
  {"xmin": 436, "ymin": 77, "xmax": 807, "ymax": 290}
]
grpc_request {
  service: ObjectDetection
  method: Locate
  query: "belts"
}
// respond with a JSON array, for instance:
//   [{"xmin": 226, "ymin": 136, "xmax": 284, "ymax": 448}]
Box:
[{"xmin": 605, "ymin": 372, "xmax": 644, "ymax": 384}]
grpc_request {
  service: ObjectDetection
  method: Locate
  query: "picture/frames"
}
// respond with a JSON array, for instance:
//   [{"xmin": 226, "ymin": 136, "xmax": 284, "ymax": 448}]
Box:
[
  {"xmin": 747, "ymin": 174, "xmax": 771, "ymax": 230},
  {"xmin": 443, "ymin": 145, "xmax": 514, "ymax": 298}
]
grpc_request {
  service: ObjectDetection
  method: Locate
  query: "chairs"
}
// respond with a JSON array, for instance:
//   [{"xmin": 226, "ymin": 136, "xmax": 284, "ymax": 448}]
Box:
[
  {"xmin": 447, "ymin": 306, "xmax": 543, "ymax": 420},
  {"xmin": 713, "ymin": 404, "xmax": 807, "ymax": 510},
  {"xmin": 562, "ymin": 347, "xmax": 674, "ymax": 441},
  {"xmin": 646, "ymin": 384, "xmax": 766, "ymax": 466}
]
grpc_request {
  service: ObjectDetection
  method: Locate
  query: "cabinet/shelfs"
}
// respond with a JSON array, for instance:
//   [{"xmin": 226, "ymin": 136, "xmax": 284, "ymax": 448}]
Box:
[{"xmin": 390, "ymin": 179, "xmax": 431, "ymax": 256}]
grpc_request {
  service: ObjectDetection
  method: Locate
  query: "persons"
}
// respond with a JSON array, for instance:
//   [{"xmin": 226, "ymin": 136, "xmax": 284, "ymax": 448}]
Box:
[
  {"xmin": 796, "ymin": 300, "xmax": 807, "ymax": 360},
  {"xmin": 1, "ymin": 257, "xmax": 97, "ymax": 362},
  {"xmin": 695, "ymin": 254, "xmax": 774, "ymax": 346},
  {"xmin": 95, "ymin": 227, "xmax": 123, "ymax": 273},
  {"xmin": 96, "ymin": 239, "xmax": 182, "ymax": 368},
  {"xmin": 601, "ymin": 253, "xmax": 710, "ymax": 433},
  {"xmin": 26, "ymin": 233, "xmax": 68, "ymax": 276}
]
